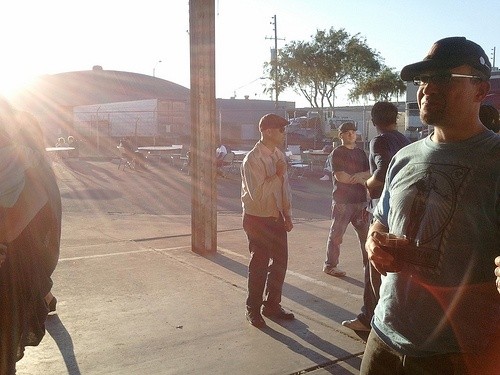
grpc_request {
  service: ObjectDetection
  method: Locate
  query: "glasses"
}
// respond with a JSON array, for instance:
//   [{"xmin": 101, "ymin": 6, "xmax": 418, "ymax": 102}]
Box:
[
  {"xmin": 413, "ymin": 71, "xmax": 482, "ymax": 86},
  {"xmin": 270, "ymin": 127, "xmax": 285, "ymax": 133}
]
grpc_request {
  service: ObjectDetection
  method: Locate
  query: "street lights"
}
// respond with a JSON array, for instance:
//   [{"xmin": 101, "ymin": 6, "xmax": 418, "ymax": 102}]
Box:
[{"xmin": 153, "ymin": 60, "xmax": 162, "ymax": 99}]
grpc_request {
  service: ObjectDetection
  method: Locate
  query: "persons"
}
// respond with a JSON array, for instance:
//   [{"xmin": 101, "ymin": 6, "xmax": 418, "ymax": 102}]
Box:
[
  {"xmin": 479, "ymin": 105, "xmax": 500, "ymax": 135},
  {"xmin": 0, "ymin": 99, "xmax": 62, "ymax": 375},
  {"xmin": 341, "ymin": 101, "xmax": 411, "ymax": 331},
  {"xmin": 56, "ymin": 136, "xmax": 78, "ymax": 160},
  {"xmin": 216, "ymin": 138, "xmax": 230, "ymax": 176},
  {"xmin": 241, "ymin": 113, "xmax": 294, "ymax": 327},
  {"xmin": 360, "ymin": 37, "xmax": 500, "ymax": 375},
  {"xmin": 323, "ymin": 123, "xmax": 370, "ymax": 277}
]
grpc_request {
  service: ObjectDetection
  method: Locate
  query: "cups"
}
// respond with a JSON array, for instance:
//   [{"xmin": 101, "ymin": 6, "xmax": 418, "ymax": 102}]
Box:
[{"xmin": 379, "ymin": 233, "xmax": 408, "ymax": 274}]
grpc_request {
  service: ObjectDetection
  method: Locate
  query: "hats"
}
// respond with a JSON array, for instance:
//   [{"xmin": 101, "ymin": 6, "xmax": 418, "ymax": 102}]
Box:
[
  {"xmin": 339, "ymin": 122, "xmax": 358, "ymax": 134},
  {"xmin": 401, "ymin": 37, "xmax": 491, "ymax": 81},
  {"xmin": 259, "ymin": 113, "xmax": 290, "ymax": 132}
]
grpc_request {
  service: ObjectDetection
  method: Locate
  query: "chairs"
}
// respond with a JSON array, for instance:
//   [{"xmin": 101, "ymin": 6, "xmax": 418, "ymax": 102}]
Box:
[
  {"xmin": 117, "ymin": 140, "xmax": 227, "ymax": 175},
  {"xmin": 289, "ymin": 154, "xmax": 309, "ymax": 179}
]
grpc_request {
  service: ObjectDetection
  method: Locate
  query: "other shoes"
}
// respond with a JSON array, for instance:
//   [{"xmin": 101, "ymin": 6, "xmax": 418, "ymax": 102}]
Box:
[
  {"xmin": 262, "ymin": 305, "xmax": 294, "ymax": 320},
  {"xmin": 244, "ymin": 306, "xmax": 266, "ymax": 327}
]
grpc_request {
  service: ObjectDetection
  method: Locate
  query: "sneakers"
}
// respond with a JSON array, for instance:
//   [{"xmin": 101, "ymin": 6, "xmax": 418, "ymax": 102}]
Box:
[
  {"xmin": 323, "ymin": 267, "xmax": 345, "ymax": 276},
  {"xmin": 342, "ymin": 318, "xmax": 370, "ymax": 331}
]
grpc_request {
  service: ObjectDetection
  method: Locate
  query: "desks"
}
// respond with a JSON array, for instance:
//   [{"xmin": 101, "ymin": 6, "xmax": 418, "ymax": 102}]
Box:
[
  {"xmin": 138, "ymin": 147, "xmax": 183, "ymax": 169},
  {"xmin": 232, "ymin": 151, "xmax": 248, "ymax": 163},
  {"xmin": 304, "ymin": 150, "xmax": 329, "ymax": 171},
  {"xmin": 46, "ymin": 147, "xmax": 75, "ymax": 163}
]
